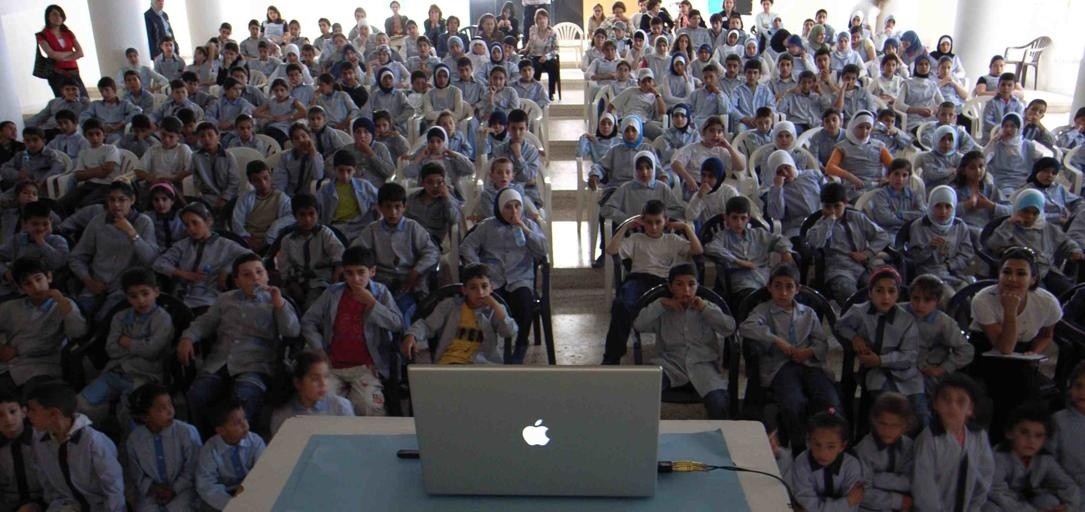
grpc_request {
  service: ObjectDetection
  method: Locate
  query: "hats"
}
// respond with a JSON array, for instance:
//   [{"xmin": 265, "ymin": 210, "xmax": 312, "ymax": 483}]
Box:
[
  {"xmin": 637, "ymin": 68, "xmax": 654, "ymax": 81},
  {"xmin": 612, "ymin": 22, "xmax": 625, "ymax": 31}
]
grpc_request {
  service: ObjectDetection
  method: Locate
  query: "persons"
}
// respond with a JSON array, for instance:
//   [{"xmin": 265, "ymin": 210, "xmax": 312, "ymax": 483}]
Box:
[
  {"xmin": 0, "ymin": 1, "xmax": 560, "ymax": 511},
  {"xmin": 578, "ymin": 1, "xmax": 1085, "ymax": 512}
]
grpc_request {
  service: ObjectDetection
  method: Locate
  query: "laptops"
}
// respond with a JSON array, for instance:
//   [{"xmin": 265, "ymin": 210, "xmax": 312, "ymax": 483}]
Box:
[{"xmin": 404, "ymin": 362, "xmax": 663, "ymax": 499}]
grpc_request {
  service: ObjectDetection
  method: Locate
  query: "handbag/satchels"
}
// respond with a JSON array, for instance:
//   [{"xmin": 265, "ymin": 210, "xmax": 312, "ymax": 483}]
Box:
[{"xmin": 32, "ymin": 32, "xmax": 54, "ymax": 79}]
